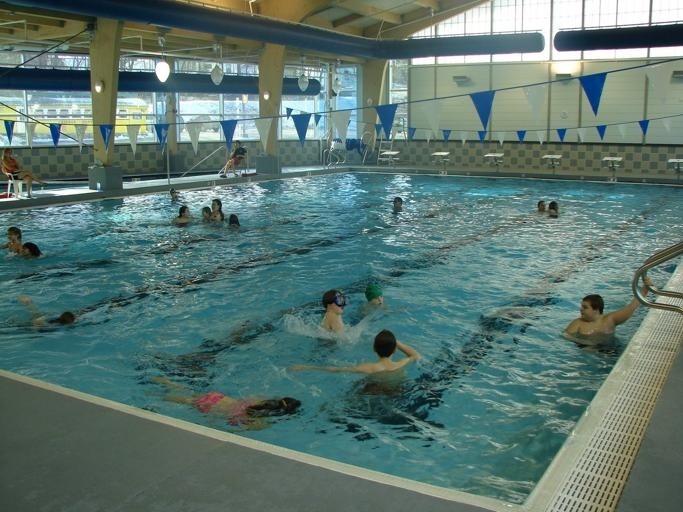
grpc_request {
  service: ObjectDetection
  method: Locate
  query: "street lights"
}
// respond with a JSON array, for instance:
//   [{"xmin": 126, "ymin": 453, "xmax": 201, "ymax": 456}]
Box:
[{"xmin": 241, "ymin": 93, "xmax": 249, "ymax": 137}]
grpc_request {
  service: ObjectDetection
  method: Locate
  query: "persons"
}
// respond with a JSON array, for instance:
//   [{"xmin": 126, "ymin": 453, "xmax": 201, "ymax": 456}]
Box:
[
  {"xmin": 320, "ymin": 290, "xmax": 348, "ymax": 343},
  {"xmin": 548, "ymin": 201, "xmax": 561, "ymax": 219},
  {"xmin": 291, "ymin": 330, "xmax": 420, "ymax": 374},
  {"xmin": 220, "ymin": 140, "xmax": 247, "ymax": 178},
  {"xmin": 561, "ymin": 277, "xmax": 654, "ymax": 353},
  {"xmin": 361, "ymin": 280, "xmax": 387, "ymax": 317},
  {"xmin": 145, "ymin": 375, "xmax": 302, "ymax": 431},
  {"xmin": 394, "ymin": 196, "xmax": 403, "ymax": 208},
  {"xmin": 19, "ymin": 292, "xmax": 75, "ymax": 331},
  {"xmin": 0, "ymin": 227, "xmax": 43, "ymax": 260},
  {"xmin": 1, "ymin": 148, "xmax": 48, "ymax": 198},
  {"xmin": 537, "ymin": 199, "xmax": 547, "ymax": 212},
  {"xmin": 169, "ymin": 186, "xmax": 241, "ymax": 229}
]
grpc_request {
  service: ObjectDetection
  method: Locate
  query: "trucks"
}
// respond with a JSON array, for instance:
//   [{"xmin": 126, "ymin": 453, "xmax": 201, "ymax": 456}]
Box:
[{"xmin": 186, "ymin": 116, "xmax": 220, "ymax": 133}]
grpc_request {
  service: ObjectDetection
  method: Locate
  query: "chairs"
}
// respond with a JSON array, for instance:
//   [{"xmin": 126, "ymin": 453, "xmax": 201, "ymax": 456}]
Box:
[
  {"xmin": 227, "ymin": 156, "xmax": 246, "ymax": 177},
  {"xmin": 3, "ymin": 171, "xmax": 34, "ymax": 199}
]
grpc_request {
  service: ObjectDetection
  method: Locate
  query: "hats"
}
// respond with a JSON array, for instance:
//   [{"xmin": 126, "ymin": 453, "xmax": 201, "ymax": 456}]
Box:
[{"xmin": 365, "ymin": 283, "xmax": 384, "ymax": 302}]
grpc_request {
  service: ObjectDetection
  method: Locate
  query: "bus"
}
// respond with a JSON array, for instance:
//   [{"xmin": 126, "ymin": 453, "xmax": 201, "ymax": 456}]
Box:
[
  {"xmin": 315, "ymin": 55, "xmax": 408, "ymax": 137},
  {"xmin": 0, "ymin": 95, "xmax": 149, "ymax": 139}
]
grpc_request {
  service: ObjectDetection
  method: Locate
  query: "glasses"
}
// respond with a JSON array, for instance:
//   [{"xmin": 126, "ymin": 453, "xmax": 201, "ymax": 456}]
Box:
[{"xmin": 334, "ymin": 295, "xmax": 350, "ymax": 307}]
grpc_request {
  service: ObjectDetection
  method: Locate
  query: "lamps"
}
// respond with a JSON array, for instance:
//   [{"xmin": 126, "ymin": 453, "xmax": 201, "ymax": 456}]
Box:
[
  {"xmin": 297, "ymin": 52, "xmax": 310, "ymax": 93},
  {"xmin": 452, "ymin": 76, "xmax": 469, "ymax": 84},
  {"xmin": 671, "ymin": 70, "xmax": 683, "ymax": 79},
  {"xmin": 153, "ymin": 30, "xmax": 170, "ymax": 83},
  {"xmin": 94, "ymin": 80, "xmax": 104, "ymax": 94},
  {"xmin": 209, "ymin": 41, "xmax": 225, "ymax": 87},
  {"xmin": 555, "ymin": 73, "xmax": 572, "ymax": 82},
  {"xmin": 263, "ymin": 89, "xmax": 271, "ymax": 102}
]
